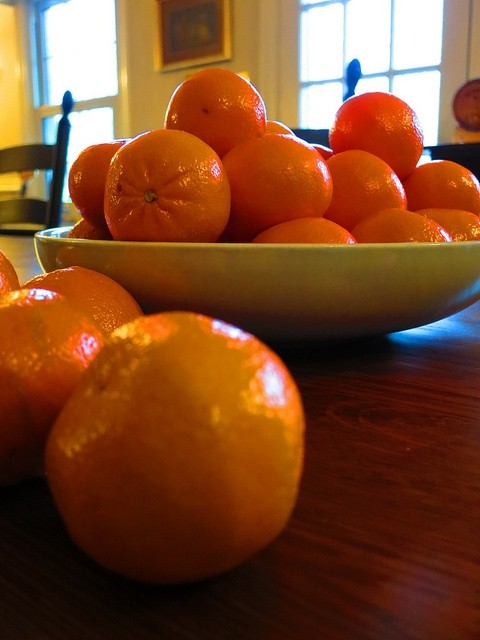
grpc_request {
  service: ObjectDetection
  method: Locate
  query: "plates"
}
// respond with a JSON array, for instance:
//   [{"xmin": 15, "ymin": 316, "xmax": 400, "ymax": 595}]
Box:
[{"xmin": 34, "ymin": 225, "xmax": 480, "ymax": 344}]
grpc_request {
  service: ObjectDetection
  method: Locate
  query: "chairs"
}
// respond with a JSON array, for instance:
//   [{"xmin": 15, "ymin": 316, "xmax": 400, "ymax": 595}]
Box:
[{"xmin": 0, "ymin": 89, "xmax": 75, "ymax": 234}]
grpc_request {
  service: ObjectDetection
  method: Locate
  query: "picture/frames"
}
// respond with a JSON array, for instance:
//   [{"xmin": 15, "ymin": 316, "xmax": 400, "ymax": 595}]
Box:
[{"xmin": 153, "ymin": 0, "xmax": 229, "ymax": 74}]
